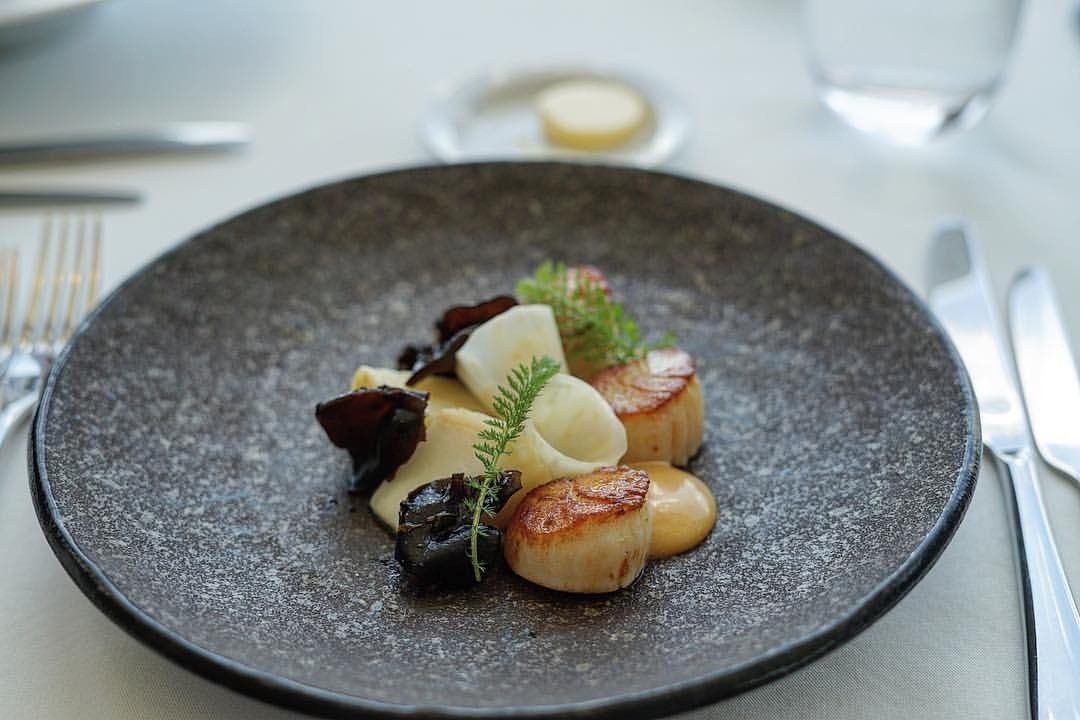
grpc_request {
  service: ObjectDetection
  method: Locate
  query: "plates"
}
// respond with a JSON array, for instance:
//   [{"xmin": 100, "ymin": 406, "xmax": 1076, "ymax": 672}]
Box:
[
  {"xmin": 0, "ymin": 0, "xmax": 93, "ymax": 25},
  {"xmin": 27, "ymin": 162, "xmax": 983, "ymax": 718},
  {"xmin": 420, "ymin": 51, "xmax": 691, "ymax": 167}
]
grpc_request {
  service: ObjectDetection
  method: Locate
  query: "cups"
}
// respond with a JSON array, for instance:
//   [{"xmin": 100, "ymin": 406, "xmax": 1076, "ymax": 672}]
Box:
[{"xmin": 801, "ymin": 0, "xmax": 1024, "ymax": 157}]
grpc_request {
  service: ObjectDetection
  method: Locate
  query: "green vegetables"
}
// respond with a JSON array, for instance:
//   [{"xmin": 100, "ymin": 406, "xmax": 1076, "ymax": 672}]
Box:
[{"xmin": 463, "ymin": 259, "xmax": 674, "ymax": 582}]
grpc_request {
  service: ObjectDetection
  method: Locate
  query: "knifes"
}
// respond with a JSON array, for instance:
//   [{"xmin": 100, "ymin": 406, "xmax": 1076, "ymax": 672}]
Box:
[
  {"xmin": 927, "ymin": 227, "xmax": 1080, "ymax": 720},
  {"xmin": 1006, "ymin": 263, "xmax": 1080, "ymax": 484}
]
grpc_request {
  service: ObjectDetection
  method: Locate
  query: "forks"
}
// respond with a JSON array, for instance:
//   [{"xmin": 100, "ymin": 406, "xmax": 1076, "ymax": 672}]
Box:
[{"xmin": 0, "ymin": 207, "xmax": 102, "ymax": 444}]
